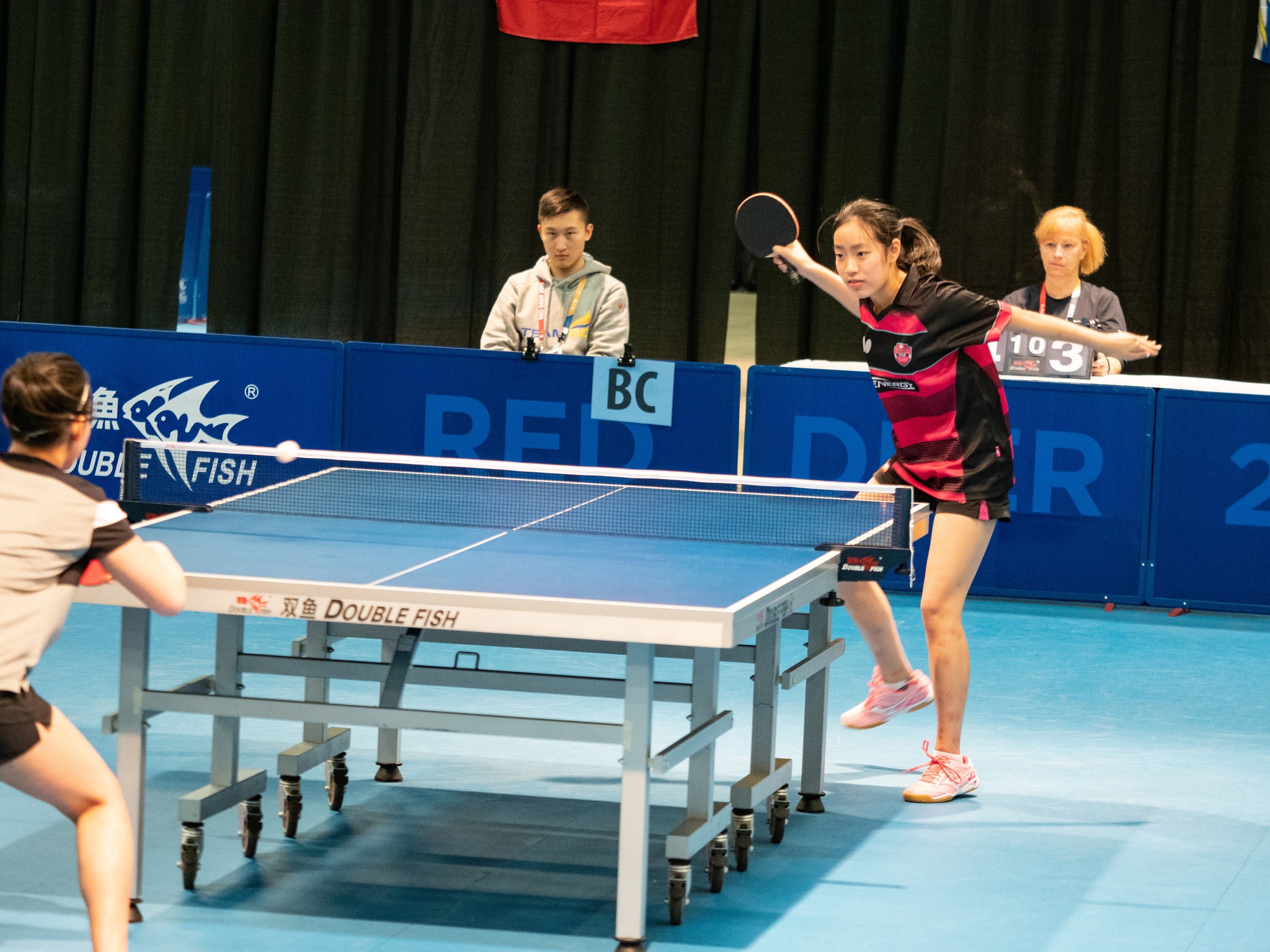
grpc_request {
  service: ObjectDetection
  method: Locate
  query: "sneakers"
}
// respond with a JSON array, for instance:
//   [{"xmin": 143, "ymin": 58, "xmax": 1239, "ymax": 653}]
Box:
[
  {"xmin": 841, "ymin": 664, "xmax": 936, "ymax": 729},
  {"xmin": 903, "ymin": 740, "xmax": 977, "ymax": 802}
]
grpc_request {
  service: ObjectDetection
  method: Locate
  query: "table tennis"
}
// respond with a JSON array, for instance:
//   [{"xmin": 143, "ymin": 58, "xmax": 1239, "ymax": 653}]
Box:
[{"xmin": 275, "ymin": 440, "xmax": 300, "ymax": 463}]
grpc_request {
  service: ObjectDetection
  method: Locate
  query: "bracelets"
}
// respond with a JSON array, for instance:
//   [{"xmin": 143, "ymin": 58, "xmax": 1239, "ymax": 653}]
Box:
[{"xmin": 1107, "ymin": 359, "xmax": 1110, "ymax": 372}]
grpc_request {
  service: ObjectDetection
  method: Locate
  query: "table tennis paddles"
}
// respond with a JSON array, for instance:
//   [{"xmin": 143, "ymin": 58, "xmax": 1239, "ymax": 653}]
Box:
[
  {"xmin": 735, "ymin": 192, "xmax": 805, "ymax": 286},
  {"xmin": 80, "ymin": 559, "xmax": 113, "ymax": 586}
]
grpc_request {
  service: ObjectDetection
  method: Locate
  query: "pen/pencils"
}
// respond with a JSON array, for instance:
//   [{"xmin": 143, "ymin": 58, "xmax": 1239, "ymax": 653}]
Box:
[{"xmin": 1094, "ymin": 350, "xmax": 1098, "ymax": 362}]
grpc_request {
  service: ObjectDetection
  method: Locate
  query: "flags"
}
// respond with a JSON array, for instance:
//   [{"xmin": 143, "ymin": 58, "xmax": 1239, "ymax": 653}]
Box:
[{"xmin": 497, "ymin": 0, "xmax": 698, "ymax": 44}]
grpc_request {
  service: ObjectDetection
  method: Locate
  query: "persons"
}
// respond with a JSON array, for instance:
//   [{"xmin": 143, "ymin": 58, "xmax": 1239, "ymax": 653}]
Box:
[
  {"xmin": 0, "ymin": 351, "xmax": 189, "ymax": 952},
  {"xmin": 1002, "ymin": 205, "xmax": 1127, "ymax": 376},
  {"xmin": 772, "ymin": 198, "xmax": 1162, "ymax": 803},
  {"xmin": 480, "ymin": 186, "xmax": 629, "ymax": 357}
]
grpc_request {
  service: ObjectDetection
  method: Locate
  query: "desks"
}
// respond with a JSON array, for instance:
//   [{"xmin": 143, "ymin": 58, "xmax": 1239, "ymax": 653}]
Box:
[{"xmin": 72, "ymin": 466, "xmax": 933, "ymax": 952}]
